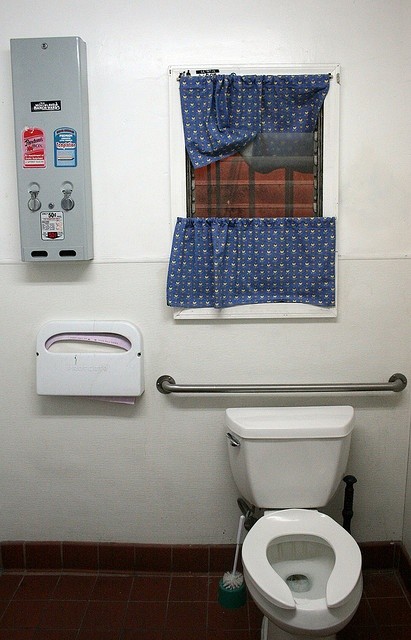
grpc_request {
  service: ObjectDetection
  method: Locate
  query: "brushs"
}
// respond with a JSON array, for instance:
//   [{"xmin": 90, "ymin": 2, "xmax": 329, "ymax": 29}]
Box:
[{"xmin": 223, "ymin": 516, "xmax": 245, "ymax": 590}]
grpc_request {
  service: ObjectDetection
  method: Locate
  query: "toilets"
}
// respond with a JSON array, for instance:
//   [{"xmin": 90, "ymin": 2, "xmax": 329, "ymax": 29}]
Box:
[{"xmin": 224, "ymin": 406, "xmax": 366, "ymax": 639}]
